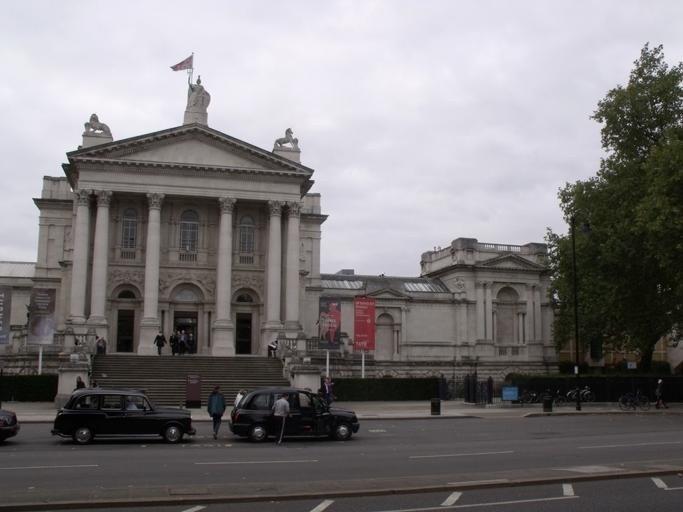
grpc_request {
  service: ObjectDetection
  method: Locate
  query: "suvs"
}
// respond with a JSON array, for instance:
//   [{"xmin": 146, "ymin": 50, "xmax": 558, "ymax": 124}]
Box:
[
  {"xmin": 228, "ymin": 387, "xmax": 359, "ymax": 443},
  {"xmin": 50, "ymin": 388, "xmax": 195, "ymax": 445}
]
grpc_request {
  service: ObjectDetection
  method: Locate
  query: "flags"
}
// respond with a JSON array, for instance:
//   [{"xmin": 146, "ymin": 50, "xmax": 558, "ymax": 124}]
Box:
[{"xmin": 170, "ymin": 55, "xmax": 192, "ymax": 71}]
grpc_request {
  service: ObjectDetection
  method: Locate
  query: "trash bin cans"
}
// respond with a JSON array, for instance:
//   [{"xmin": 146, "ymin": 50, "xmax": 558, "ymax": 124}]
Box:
[
  {"xmin": 543, "ymin": 395, "xmax": 553, "ymax": 413},
  {"xmin": 431, "ymin": 398, "xmax": 440, "ymax": 415}
]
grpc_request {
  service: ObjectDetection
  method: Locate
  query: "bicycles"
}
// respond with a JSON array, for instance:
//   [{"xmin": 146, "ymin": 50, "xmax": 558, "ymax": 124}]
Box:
[
  {"xmin": 517, "ymin": 386, "xmax": 595, "ymax": 408},
  {"xmin": 618, "ymin": 390, "xmax": 650, "ymax": 411}
]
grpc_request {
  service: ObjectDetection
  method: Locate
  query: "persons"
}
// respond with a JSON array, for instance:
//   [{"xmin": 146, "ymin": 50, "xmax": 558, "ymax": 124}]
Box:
[
  {"xmin": 321, "ymin": 375, "xmax": 337, "ymax": 405},
  {"xmin": 74, "ymin": 376, "xmax": 85, "ymax": 391},
  {"xmin": 208, "ymin": 385, "xmax": 226, "ymax": 440},
  {"xmin": 154, "ymin": 330, "xmax": 167, "ymax": 355},
  {"xmin": 170, "ymin": 329, "xmax": 195, "ymax": 356},
  {"xmin": 91, "ymin": 378, "xmax": 97, "ymax": 388},
  {"xmin": 271, "ymin": 393, "xmax": 291, "ymax": 445},
  {"xmin": 267, "ymin": 340, "xmax": 277, "ymax": 358},
  {"xmin": 125, "ymin": 398, "xmax": 139, "ymax": 410},
  {"xmin": 655, "ymin": 378, "xmax": 669, "ymax": 410},
  {"xmin": 188, "ymin": 75, "xmax": 204, "ymax": 112}
]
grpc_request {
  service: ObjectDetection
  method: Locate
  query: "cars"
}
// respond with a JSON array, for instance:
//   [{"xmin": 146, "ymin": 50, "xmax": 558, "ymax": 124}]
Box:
[{"xmin": 0, "ymin": 409, "xmax": 19, "ymax": 441}]
